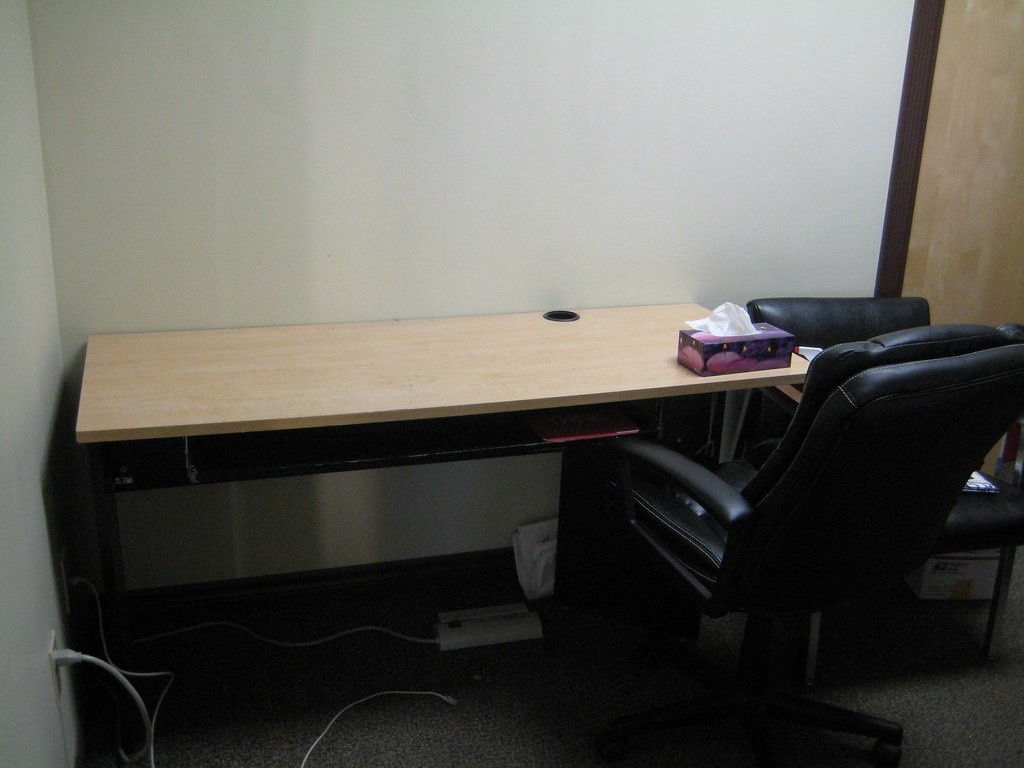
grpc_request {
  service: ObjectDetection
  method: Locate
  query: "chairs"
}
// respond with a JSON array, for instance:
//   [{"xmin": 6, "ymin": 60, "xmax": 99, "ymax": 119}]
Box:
[
  {"xmin": 599, "ymin": 322, "xmax": 1023, "ymax": 768},
  {"xmin": 746, "ymin": 297, "xmax": 1024, "ymax": 660}
]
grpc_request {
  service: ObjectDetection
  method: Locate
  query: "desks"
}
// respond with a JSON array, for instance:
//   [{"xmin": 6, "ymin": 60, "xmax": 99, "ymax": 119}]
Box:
[{"xmin": 72, "ymin": 297, "xmax": 813, "ymax": 734}]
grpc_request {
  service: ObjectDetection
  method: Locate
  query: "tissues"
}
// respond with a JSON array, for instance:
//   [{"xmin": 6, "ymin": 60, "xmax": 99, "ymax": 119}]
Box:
[{"xmin": 677, "ymin": 301, "xmax": 796, "ymax": 376}]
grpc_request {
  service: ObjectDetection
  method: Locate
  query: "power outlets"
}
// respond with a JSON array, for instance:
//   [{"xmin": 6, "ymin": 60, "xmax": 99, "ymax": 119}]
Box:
[
  {"xmin": 61, "ymin": 546, "xmax": 72, "ymax": 615},
  {"xmin": 48, "ymin": 630, "xmax": 63, "ymax": 705}
]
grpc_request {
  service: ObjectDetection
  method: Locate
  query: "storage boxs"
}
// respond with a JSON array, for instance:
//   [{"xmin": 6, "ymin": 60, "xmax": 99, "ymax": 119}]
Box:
[{"xmin": 905, "ymin": 471, "xmax": 1002, "ymax": 601}]
